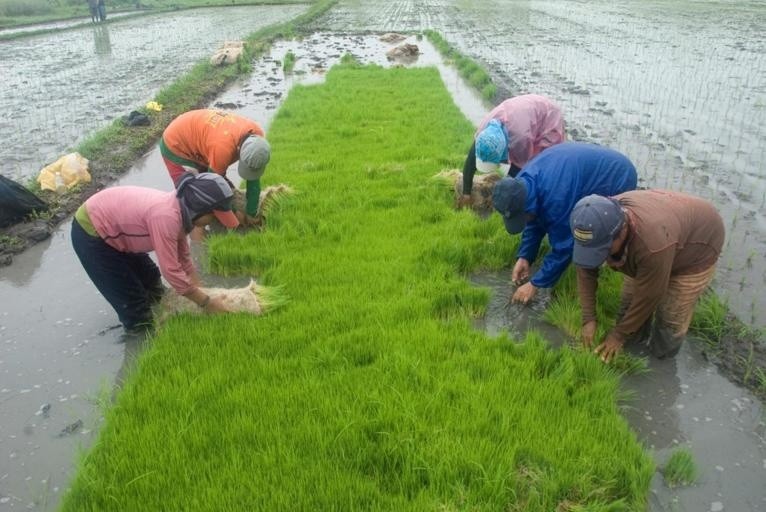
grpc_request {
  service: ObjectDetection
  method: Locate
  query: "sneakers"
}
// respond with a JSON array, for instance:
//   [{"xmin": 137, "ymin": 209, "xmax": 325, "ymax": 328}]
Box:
[{"xmin": 123, "ymin": 111, "xmax": 150, "ymax": 130}]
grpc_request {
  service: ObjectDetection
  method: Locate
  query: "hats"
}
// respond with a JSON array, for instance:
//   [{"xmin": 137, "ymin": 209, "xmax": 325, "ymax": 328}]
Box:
[
  {"xmin": 211, "ymin": 196, "xmax": 240, "ymax": 229},
  {"xmin": 238, "ymin": 135, "xmax": 270, "ymax": 181},
  {"xmin": 475, "ymin": 157, "xmax": 498, "ymax": 173},
  {"xmin": 492, "ymin": 177, "xmax": 526, "ymax": 235},
  {"xmin": 570, "ymin": 194, "xmax": 624, "ymax": 270}
]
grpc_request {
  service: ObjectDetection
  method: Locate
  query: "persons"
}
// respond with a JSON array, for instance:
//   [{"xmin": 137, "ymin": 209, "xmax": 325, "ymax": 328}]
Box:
[
  {"xmin": 569, "ymin": 191, "xmax": 725, "ymax": 362},
  {"xmin": 98, "ymin": 0, "xmax": 107, "ymax": 22},
  {"xmin": 86, "ymin": 0, "xmax": 98, "ymax": 23},
  {"xmin": 71, "ymin": 170, "xmax": 242, "ymax": 334},
  {"xmin": 161, "ymin": 108, "xmax": 273, "ymax": 238},
  {"xmin": 457, "ymin": 92, "xmax": 567, "ymax": 201},
  {"xmin": 490, "ymin": 138, "xmax": 639, "ymax": 308}
]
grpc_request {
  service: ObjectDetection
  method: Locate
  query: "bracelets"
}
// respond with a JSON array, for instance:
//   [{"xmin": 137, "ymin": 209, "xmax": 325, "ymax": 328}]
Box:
[{"xmin": 199, "ymin": 296, "xmax": 210, "ymax": 308}]
